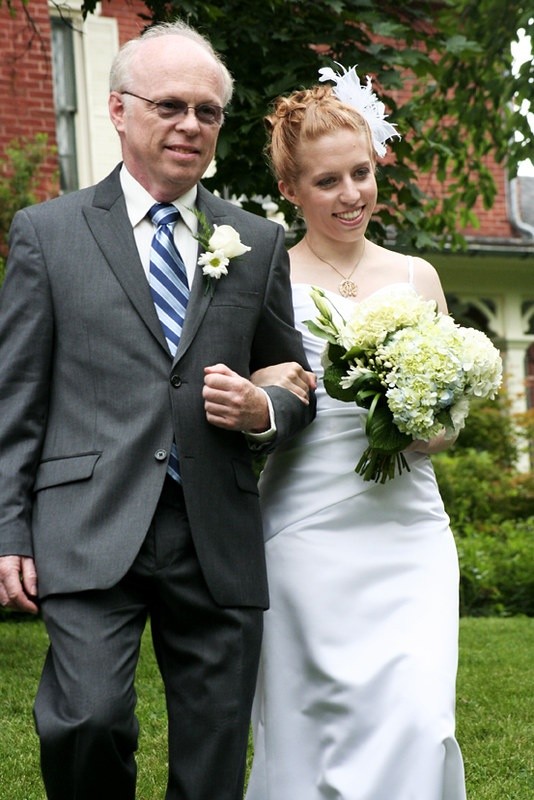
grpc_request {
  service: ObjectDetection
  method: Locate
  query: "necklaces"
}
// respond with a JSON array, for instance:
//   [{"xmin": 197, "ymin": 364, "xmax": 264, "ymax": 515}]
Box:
[{"xmin": 304, "ymin": 234, "xmax": 365, "ymax": 298}]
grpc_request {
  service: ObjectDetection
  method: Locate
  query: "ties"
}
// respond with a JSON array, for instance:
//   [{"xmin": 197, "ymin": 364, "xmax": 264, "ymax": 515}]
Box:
[{"xmin": 144, "ymin": 206, "xmax": 194, "ymax": 485}]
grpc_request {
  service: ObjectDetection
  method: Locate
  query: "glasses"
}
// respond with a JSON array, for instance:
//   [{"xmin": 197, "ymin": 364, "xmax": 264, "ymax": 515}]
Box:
[{"xmin": 121, "ymin": 90, "xmax": 228, "ymax": 127}]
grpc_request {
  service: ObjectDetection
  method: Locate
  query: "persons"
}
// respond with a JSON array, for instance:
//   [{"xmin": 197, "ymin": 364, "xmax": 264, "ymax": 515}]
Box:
[
  {"xmin": 245, "ymin": 86, "xmax": 467, "ymax": 800},
  {"xmin": 0, "ymin": 16, "xmax": 316, "ymax": 800}
]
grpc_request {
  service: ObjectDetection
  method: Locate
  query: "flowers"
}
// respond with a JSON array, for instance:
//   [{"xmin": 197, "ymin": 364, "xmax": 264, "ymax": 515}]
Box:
[
  {"xmin": 318, "ymin": 61, "xmax": 401, "ymax": 158},
  {"xmin": 301, "ymin": 283, "xmax": 505, "ymax": 484},
  {"xmin": 185, "ymin": 205, "xmax": 251, "ymax": 298}
]
